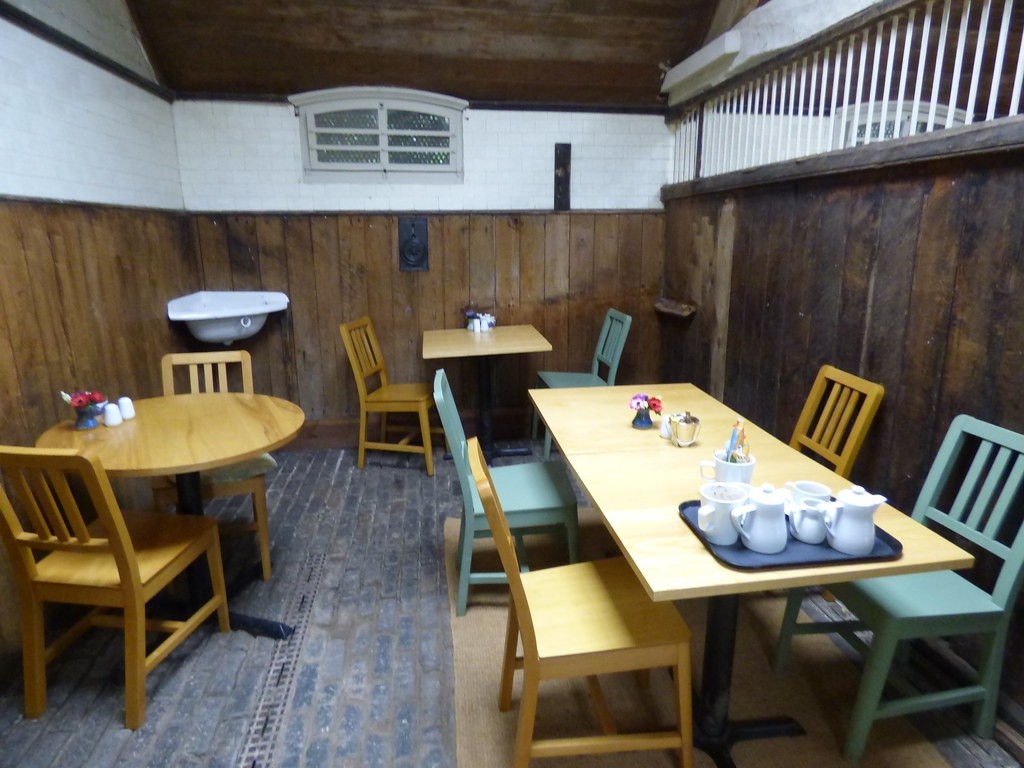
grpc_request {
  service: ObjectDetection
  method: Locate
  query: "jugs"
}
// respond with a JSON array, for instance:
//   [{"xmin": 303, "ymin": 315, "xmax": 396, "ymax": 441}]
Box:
[
  {"xmin": 822, "ymin": 486, "xmax": 887, "ymax": 555},
  {"xmin": 731, "ymin": 484, "xmax": 792, "ymax": 554}
]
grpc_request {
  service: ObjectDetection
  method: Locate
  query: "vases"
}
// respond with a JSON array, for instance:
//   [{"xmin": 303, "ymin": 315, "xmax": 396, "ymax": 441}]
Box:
[
  {"xmin": 76, "ymin": 407, "xmax": 104, "ymax": 429},
  {"xmin": 633, "ymin": 409, "xmax": 653, "ymax": 429}
]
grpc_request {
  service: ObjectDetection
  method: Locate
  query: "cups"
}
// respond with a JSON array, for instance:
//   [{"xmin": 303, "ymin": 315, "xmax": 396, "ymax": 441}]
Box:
[
  {"xmin": 698, "ymin": 483, "xmax": 747, "ymax": 546},
  {"xmin": 788, "ymin": 498, "xmax": 831, "ymax": 544},
  {"xmin": 784, "ymin": 482, "xmax": 831, "ymax": 505},
  {"xmin": 699, "ymin": 450, "xmax": 756, "ymax": 484}
]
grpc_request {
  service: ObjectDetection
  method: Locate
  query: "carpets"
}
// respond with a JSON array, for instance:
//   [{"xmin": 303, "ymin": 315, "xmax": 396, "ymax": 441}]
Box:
[{"xmin": 446, "ymin": 518, "xmax": 954, "ymax": 768}]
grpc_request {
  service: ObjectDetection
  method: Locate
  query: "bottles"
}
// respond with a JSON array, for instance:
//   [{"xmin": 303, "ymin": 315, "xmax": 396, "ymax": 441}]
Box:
[
  {"xmin": 118, "ymin": 396, "xmax": 135, "ymax": 420},
  {"xmin": 660, "ymin": 411, "xmax": 699, "ymax": 446},
  {"xmin": 103, "ymin": 404, "xmax": 121, "ymax": 427},
  {"xmin": 473, "ymin": 312, "xmax": 495, "ymax": 333}
]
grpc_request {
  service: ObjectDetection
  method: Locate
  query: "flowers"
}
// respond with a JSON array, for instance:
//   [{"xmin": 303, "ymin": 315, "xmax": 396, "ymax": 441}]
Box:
[
  {"xmin": 71, "ymin": 391, "xmax": 105, "ymax": 408},
  {"xmin": 630, "ymin": 393, "xmax": 662, "ymax": 415}
]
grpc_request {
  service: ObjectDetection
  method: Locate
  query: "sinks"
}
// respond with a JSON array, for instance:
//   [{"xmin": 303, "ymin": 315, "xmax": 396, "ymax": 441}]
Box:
[{"xmin": 167, "ymin": 290, "xmax": 290, "ymax": 345}]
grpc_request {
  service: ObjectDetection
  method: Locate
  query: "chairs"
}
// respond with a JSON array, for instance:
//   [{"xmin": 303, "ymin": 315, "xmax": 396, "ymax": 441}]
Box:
[
  {"xmin": 532, "ymin": 307, "xmax": 631, "ymax": 460},
  {"xmin": 338, "ymin": 316, "xmax": 452, "ymax": 478},
  {"xmin": 0, "ymin": 445, "xmax": 232, "ymax": 725},
  {"xmin": 467, "ymin": 436, "xmax": 693, "ymax": 768},
  {"xmin": 789, "ymin": 364, "xmax": 885, "ymax": 601},
  {"xmin": 773, "ymin": 414, "xmax": 1024, "ymax": 763},
  {"xmin": 433, "ymin": 367, "xmax": 582, "ymax": 616},
  {"xmin": 151, "ymin": 349, "xmax": 272, "ymax": 581}
]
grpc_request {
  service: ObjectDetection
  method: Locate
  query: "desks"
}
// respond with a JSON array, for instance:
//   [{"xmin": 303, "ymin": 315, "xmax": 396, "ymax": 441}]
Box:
[
  {"xmin": 422, "ymin": 324, "xmax": 554, "ymax": 464},
  {"xmin": 34, "ymin": 393, "xmax": 306, "ymax": 625},
  {"xmin": 526, "ymin": 383, "xmax": 974, "ymax": 768}
]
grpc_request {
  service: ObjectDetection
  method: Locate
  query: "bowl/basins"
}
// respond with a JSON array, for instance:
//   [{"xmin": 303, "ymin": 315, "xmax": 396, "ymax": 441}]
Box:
[{"xmin": 94, "ymin": 399, "xmax": 108, "ymax": 415}]
